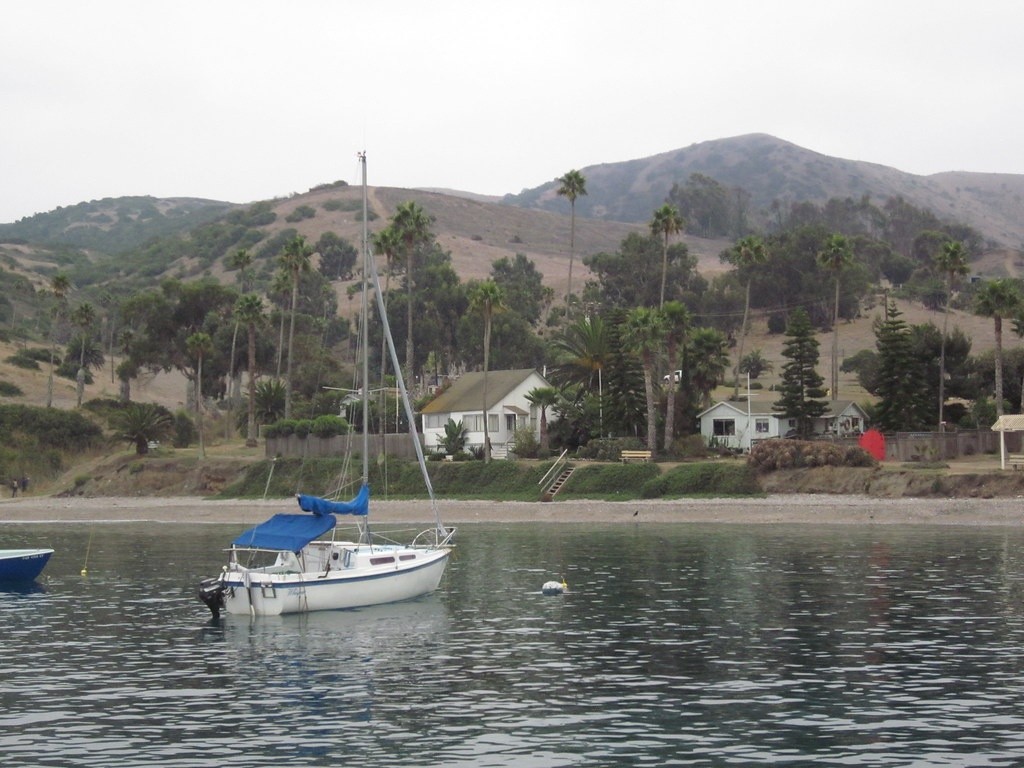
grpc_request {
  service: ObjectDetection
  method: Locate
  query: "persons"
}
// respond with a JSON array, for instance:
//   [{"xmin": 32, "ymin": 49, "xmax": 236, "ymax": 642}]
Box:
[
  {"xmin": 12, "ymin": 478, "xmax": 18, "ymax": 498},
  {"xmin": 21, "ymin": 474, "xmax": 26, "ymax": 496}
]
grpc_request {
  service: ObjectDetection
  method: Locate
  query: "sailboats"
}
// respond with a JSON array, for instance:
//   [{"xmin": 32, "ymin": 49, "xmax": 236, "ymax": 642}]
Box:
[{"xmin": 207, "ymin": 141, "xmax": 456, "ymax": 618}]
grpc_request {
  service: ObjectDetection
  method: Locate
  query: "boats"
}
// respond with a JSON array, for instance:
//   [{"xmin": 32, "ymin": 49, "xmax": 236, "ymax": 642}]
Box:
[{"xmin": 1, "ymin": 545, "xmax": 53, "ymax": 585}]
